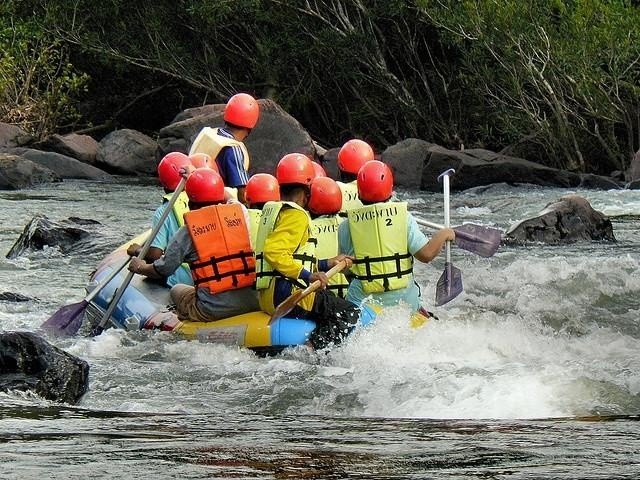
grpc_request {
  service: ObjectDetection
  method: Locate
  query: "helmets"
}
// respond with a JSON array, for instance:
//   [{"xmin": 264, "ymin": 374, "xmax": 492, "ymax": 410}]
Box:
[
  {"xmin": 355, "ymin": 159, "xmax": 395, "ymax": 202},
  {"xmin": 155, "ymin": 151, "xmax": 193, "ymax": 193},
  {"xmin": 189, "ymin": 152, "xmax": 222, "ymax": 174},
  {"xmin": 243, "ymin": 172, "xmax": 282, "ymax": 207},
  {"xmin": 274, "ymin": 150, "xmax": 314, "ymax": 188},
  {"xmin": 308, "ymin": 175, "xmax": 345, "ymax": 218},
  {"xmin": 309, "ymin": 161, "xmax": 328, "ymax": 182},
  {"xmin": 335, "ymin": 138, "xmax": 376, "ymax": 175},
  {"xmin": 185, "ymin": 166, "xmax": 226, "ymax": 207},
  {"xmin": 220, "ymin": 90, "xmax": 261, "ymax": 131}
]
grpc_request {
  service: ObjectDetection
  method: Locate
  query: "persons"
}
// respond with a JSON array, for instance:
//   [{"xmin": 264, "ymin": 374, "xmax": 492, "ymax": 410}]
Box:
[
  {"xmin": 190, "ymin": 93, "xmax": 260, "ymax": 204},
  {"xmin": 189, "ymin": 153, "xmax": 239, "ymax": 202},
  {"xmin": 337, "ymin": 160, "xmax": 456, "ymax": 316},
  {"xmin": 336, "ymin": 139, "xmax": 374, "ymax": 216},
  {"xmin": 305, "ymin": 176, "xmax": 349, "ymax": 297},
  {"xmin": 243, "ymin": 173, "xmax": 280, "ymax": 252},
  {"xmin": 256, "ymin": 152, "xmax": 361, "ymax": 354},
  {"xmin": 126, "ymin": 152, "xmax": 195, "ymax": 287},
  {"xmin": 127, "ymin": 165, "xmax": 262, "ymax": 323},
  {"xmin": 311, "ymin": 161, "xmax": 327, "ymax": 180}
]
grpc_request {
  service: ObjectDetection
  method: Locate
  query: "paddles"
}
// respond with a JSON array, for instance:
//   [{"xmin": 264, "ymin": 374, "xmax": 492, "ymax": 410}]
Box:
[
  {"xmin": 411, "ymin": 217, "xmax": 504, "ymax": 258},
  {"xmin": 435, "ymin": 169, "xmax": 463, "ymax": 307},
  {"xmin": 267, "ymin": 259, "xmax": 351, "ymax": 324},
  {"xmin": 40, "ymin": 233, "xmax": 152, "ymax": 335}
]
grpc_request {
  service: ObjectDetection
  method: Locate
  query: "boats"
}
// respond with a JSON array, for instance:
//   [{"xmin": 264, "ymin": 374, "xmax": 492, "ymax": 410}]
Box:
[{"xmin": 81, "ymin": 227, "xmax": 425, "ymax": 360}]
